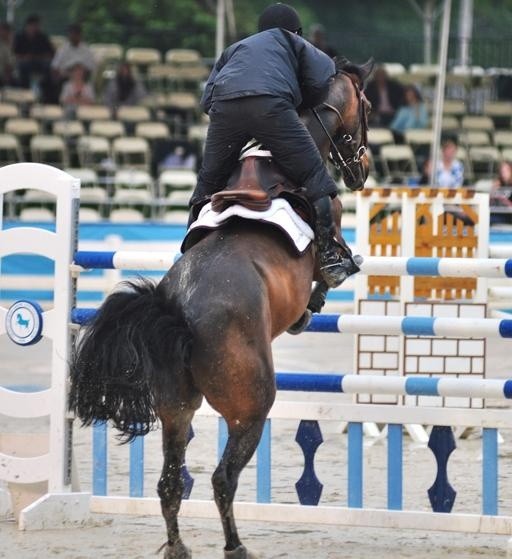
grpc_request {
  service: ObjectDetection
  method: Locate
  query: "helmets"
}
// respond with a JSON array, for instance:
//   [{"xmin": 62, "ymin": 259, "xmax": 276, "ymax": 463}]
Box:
[{"xmin": 258, "ymin": 2, "xmax": 303, "ymax": 33}]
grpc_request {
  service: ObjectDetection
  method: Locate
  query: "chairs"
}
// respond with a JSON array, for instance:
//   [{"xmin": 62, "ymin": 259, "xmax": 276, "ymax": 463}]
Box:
[
  {"xmin": 337, "ymin": 63, "xmax": 511, "ymax": 213},
  {"xmin": 1, "ymin": 32, "xmax": 210, "ymax": 220}
]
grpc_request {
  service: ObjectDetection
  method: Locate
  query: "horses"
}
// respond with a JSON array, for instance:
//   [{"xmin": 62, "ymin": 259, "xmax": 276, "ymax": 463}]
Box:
[{"xmin": 66, "ymin": 46, "xmax": 376, "ymax": 559}]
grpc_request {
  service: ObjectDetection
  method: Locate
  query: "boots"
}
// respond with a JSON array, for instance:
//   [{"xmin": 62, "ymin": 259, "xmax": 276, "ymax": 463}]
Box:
[{"xmin": 311, "ymin": 197, "xmax": 363, "ymax": 290}]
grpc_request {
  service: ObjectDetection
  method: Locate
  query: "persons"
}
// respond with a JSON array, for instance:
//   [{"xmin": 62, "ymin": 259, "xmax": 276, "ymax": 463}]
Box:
[
  {"xmin": 490, "ymin": 158, "xmax": 512, "ymax": 207},
  {"xmin": 160, "ymin": 144, "xmax": 197, "ymax": 170},
  {"xmin": 367, "ymin": 67, "xmax": 405, "ymax": 125},
  {"xmin": 58, "ymin": 63, "xmax": 97, "ymax": 105},
  {"xmin": 420, "ymin": 132, "xmax": 467, "ymax": 187},
  {"xmin": 307, "ymin": 20, "xmax": 337, "ymax": 57},
  {"xmin": 391, "ymin": 84, "xmax": 429, "ymax": 129},
  {"xmin": 186, "ymin": 2, "xmax": 364, "ymax": 288},
  {"xmin": 12, "ymin": 16, "xmax": 53, "ymax": 88},
  {"xmin": 53, "ymin": 22, "xmax": 96, "ymax": 72},
  {"xmin": 103, "ymin": 62, "xmax": 145, "ymax": 106}
]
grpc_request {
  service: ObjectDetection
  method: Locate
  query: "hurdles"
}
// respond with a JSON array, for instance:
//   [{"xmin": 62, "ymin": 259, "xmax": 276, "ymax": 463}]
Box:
[{"xmin": 71, "ymin": 250, "xmax": 512, "ymax": 534}]
